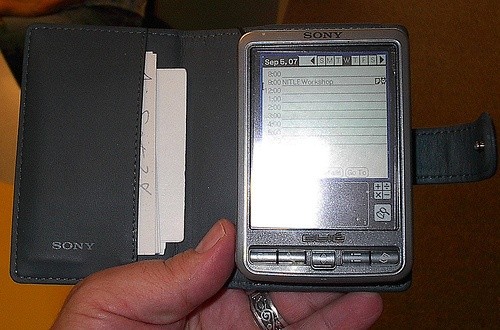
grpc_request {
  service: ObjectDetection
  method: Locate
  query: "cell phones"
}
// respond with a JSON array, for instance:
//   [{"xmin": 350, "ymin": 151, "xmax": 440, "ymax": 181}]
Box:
[{"xmin": 238, "ymin": 28, "xmax": 410, "ymax": 286}]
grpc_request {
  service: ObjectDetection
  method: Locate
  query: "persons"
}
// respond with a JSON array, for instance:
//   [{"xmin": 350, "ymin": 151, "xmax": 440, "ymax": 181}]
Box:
[{"xmin": 49, "ymin": 219, "xmax": 384, "ymax": 330}]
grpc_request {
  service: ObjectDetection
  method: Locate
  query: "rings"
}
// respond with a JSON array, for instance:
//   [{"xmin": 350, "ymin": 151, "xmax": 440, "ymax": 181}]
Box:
[{"xmin": 249, "ymin": 289, "xmax": 289, "ymax": 330}]
